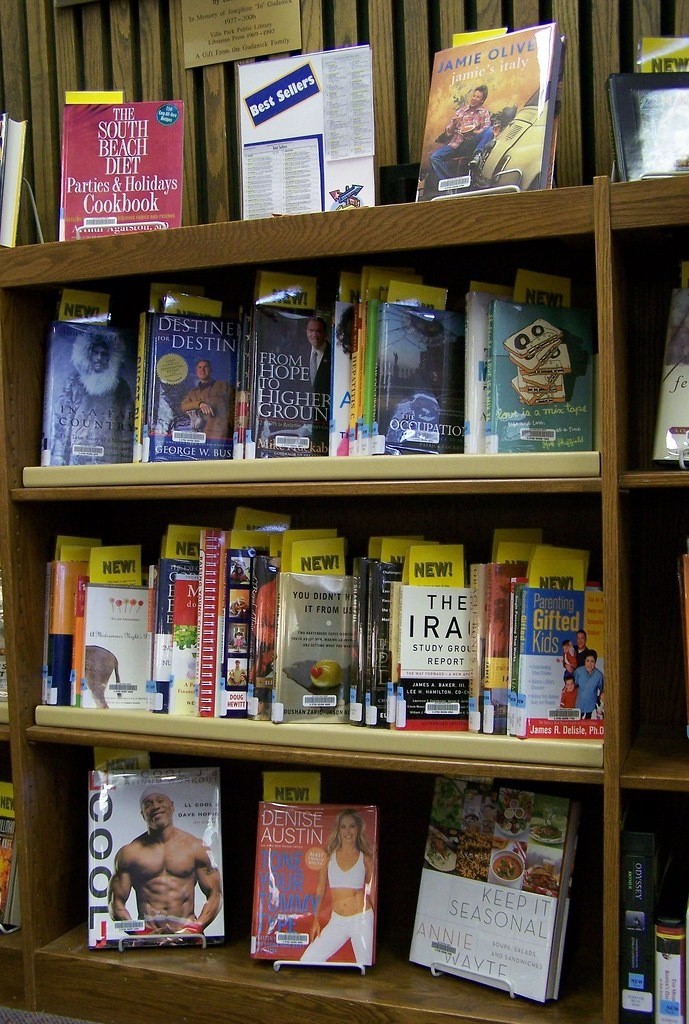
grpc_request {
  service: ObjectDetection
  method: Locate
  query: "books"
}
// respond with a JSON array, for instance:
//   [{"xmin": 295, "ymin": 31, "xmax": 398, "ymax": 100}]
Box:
[
  {"xmin": 602, "ymin": 38, "xmax": 689, "ymax": 181},
  {"xmin": 237, "ymin": 43, "xmax": 376, "ymax": 221},
  {"xmin": 620, "ymin": 812, "xmax": 687, "ymax": 1024},
  {"xmin": 416, "ymin": 23, "xmax": 565, "ymax": 202},
  {"xmin": 649, "ymin": 261, "xmax": 689, "ymax": 468},
  {"xmin": 409, "ymin": 776, "xmax": 586, "ymax": 1004},
  {"xmin": 85, "ymin": 766, "xmax": 228, "ymax": 947},
  {"xmin": 0, "ymin": 113, "xmax": 29, "ymax": 249},
  {"xmin": 41, "ymin": 510, "xmax": 607, "ymax": 738},
  {"xmin": 38, "ymin": 269, "xmax": 597, "ymax": 452}
]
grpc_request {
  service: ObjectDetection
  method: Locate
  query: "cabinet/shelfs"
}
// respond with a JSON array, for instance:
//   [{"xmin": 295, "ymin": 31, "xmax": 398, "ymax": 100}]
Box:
[{"xmin": 0, "ymin": 176, "xmax": 689, "ymax": 1024}]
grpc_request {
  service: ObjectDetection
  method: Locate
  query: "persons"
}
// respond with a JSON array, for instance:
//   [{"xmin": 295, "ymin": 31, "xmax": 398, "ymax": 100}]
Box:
[
  {"xmin": 182, "ymin": 359, "xmax": 237, "ymax": 436},
  {"xmin": 594, "ymin": 691, "xmax": 605, "ymax": 719},
  {"xmin": 304, "ymin": 810, "xmax": 375, "ymax": 965},
  {"xmin": 559, "ymin": 674, "xmax": 578, "ymax": 715},
  {"xmin": 429, "ymin": 84, "xmax": 495, "ymax": 192},
  {"xmin": 107, "ymin": 786, "xmax": 222, "ymax": 946},
  {"xmin": 554, "ymin": 640, "xmax": 577, "ymax": 677},
  {"xmin": 561, "ymin": 630, "xmax": 590, "ymax": 679},
  {"xmin": 52, "ymin": 328, "xmax": 134, "ymax": 465},
  {"xmin": 574, "ymin": 649, "xmax": 606, "ymax": 719},
  {"xmin": 288, "ymin": 319, "xmax": 331, "ymax": 450}
]
